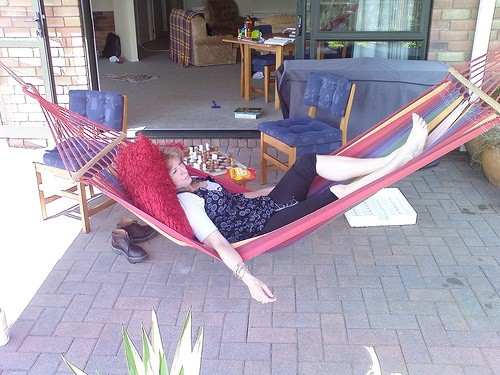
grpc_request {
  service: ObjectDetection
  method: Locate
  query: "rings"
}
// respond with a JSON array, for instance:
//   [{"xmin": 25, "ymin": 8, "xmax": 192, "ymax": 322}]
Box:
[{"xmin": 259, "ymin": 300, "xmax": 263, "ymax": 303}]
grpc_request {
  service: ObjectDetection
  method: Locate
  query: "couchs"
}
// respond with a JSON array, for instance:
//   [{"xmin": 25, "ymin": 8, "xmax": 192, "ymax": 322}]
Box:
[{"xmin": 169, "ymin": 8, "xmax": 237, "ymax": 67}]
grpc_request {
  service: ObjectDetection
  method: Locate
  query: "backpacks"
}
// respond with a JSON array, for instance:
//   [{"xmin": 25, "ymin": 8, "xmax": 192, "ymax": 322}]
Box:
[{"xmin": 103, "ymin": 32, "xmax": 121, "ymax": 58}]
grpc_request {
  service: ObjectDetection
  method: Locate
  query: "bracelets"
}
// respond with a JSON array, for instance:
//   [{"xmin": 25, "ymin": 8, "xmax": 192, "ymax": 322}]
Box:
[{"xmin": 235, "ymin": 262, "xmax": 248, "ymax": 280}]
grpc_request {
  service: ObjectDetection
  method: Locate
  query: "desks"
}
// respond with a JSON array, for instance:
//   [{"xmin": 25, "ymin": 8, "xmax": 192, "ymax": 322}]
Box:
[
  {"xmin": 275, "ymin": 57, "xmax": 470, "ymax": 169},
  {"xmin": 221, "ymin": 34, "xmax": 315, "ymax": 109}
]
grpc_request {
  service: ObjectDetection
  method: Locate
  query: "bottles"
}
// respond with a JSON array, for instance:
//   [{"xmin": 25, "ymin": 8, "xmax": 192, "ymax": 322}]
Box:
[{"xmin": 244, "ymin": 15, "xmax": 252, "ymax": 38}]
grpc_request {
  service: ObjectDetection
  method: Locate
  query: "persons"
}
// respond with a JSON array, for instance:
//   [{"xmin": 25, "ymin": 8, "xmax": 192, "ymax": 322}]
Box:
[{"xmin": 158, "ymin": 113, "xmax": 428, "ymax": 304}]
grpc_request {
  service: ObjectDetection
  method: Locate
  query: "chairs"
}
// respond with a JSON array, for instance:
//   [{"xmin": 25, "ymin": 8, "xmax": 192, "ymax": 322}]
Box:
[
  {"xmin": 238, "ymin": 25, "xmax": 295, "ymax": 103},
  {"xmin": 32, "ymin": 89, "xmax": 128, "ymax": 234},
  {"xmin": 259, "ymin": 71, "xmax": 356, "ymax": 186}
]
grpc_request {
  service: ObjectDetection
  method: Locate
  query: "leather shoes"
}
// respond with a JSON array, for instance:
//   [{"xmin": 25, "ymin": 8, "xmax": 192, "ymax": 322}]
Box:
[
  {"xmin": 111, "ymin": 228, "xmax": 148, "ymax": 264},
  {"xmin": 117, "ymin": 218, "xmax": 157, "ymax": 243}
]
